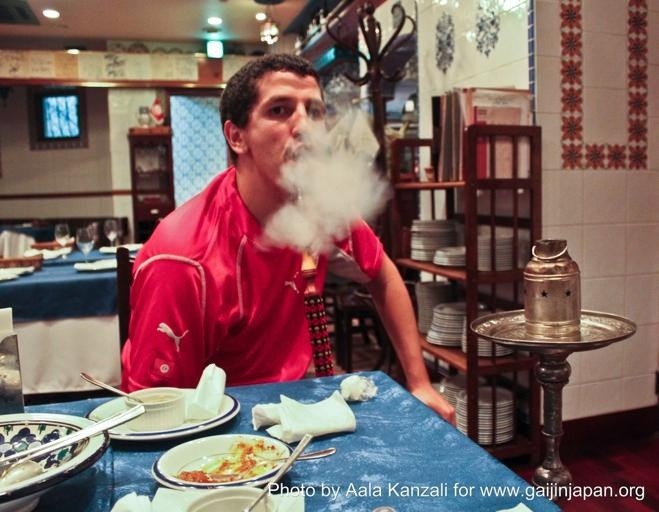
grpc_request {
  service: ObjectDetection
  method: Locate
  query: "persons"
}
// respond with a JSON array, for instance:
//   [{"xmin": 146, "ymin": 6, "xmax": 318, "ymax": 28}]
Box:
[{"xmin": 118, "ymin": 51, "xmax": 456, "ymax": 429}]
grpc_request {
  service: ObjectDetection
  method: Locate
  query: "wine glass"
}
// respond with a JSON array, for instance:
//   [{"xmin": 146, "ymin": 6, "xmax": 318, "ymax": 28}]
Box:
[
  {"xmin": 75, "ymin": 226, "xmax": 96, "ymax": 262},
  {"xmin": 54, "ymin": 224, "xmax": 70, "ymax": 259},
  {"xmin": 104, "ymin": 219, "xmax": 119, "ymax": 248}
]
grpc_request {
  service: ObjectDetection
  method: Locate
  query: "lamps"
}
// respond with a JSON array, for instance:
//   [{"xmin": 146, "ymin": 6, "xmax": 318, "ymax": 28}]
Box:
[{"xmin": 254, "ymin": 0, "xmax": 284, "ymax": 45}]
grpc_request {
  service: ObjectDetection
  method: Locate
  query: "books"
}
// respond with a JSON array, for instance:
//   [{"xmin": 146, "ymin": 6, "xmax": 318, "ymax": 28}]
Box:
[{"xmin": 431, "ymin": 86, "xmax": 531, "ymax": 182}]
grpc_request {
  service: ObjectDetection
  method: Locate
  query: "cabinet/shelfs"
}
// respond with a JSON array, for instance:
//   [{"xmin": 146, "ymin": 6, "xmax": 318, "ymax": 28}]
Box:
[
  {"xmin": 391, "ymin": 124, "xmax": 541, "ymax": 466},
  {"xmin": 128, "ymin": 126, "xmax": 175, "ymax": 243}
]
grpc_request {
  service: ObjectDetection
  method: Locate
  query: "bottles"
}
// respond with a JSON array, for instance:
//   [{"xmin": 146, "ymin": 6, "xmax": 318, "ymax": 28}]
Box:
[
  {"xmin": 146, "ymin": 207, "xmax": 159, "ymax": 238},
  {"xmin": 138, "ymin": 98, "xmax": 164, "ymax": 128}
]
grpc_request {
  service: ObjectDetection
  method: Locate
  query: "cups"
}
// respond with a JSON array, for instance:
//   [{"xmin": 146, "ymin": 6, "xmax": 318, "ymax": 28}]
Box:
[
  {"xmin": 123, "ymin": 387, "xmax": 187, "ymax": 433},
  {"xmin": 186, "ymin": 484, "xmax": 278, "ymax": 512}
]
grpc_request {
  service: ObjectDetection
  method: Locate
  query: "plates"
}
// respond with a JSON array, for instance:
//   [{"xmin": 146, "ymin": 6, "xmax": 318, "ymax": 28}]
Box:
[
  {"xmin": 99, "ymin": 242, "xmax": 143, "ymax": 255},
  {"xmin": 406, "ymin": 216, "xmax": 518, "ymax": 446},
  {"xmin": 151, "ymin": 433, "xmax": 296, "ymax": 492},
  {"xmin": 84, "ymin": 386, "xmax": 240, "ymax": 440},
  {"xmin": 74, "ymin": 259, "xmax": 117, "ymax": 271},
  {"xmin": 111, "ymin": 486, "xmax": 216, "ymax": 512}
]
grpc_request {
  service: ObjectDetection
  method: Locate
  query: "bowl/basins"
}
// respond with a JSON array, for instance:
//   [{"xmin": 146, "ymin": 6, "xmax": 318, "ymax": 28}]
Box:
[{"xmin": 0, "ymin": 413, "xmax": 112, "ymax": 511}]
